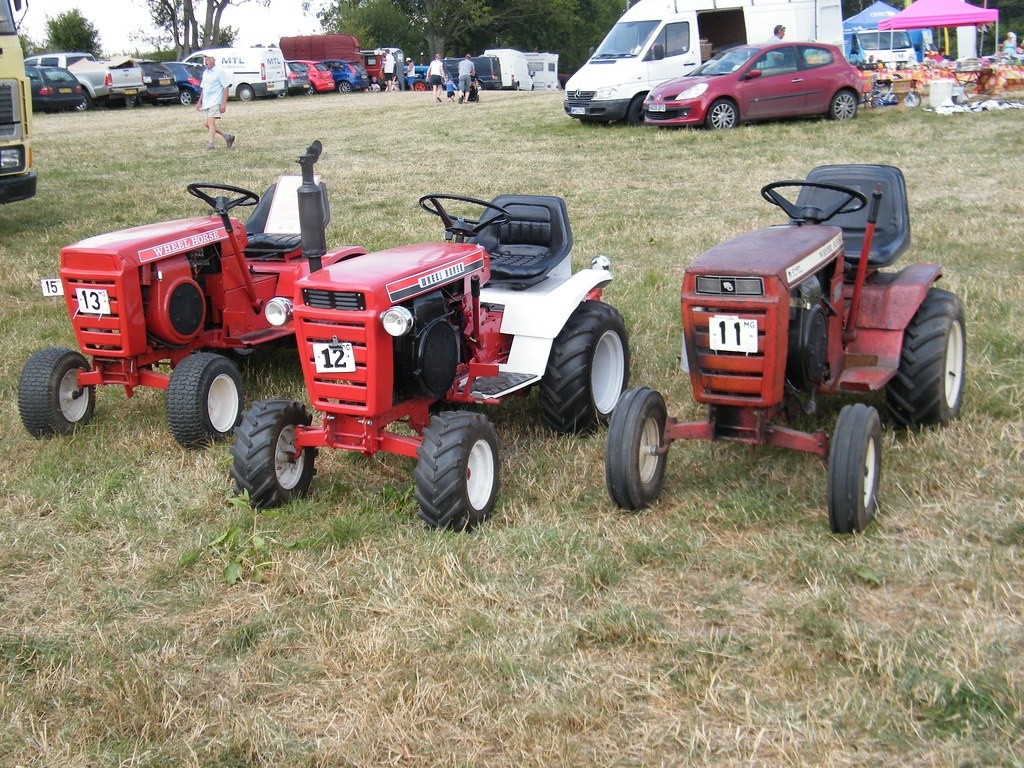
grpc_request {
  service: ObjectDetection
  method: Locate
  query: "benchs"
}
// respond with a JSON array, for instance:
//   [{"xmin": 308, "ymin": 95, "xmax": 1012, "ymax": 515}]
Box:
[{"xmin": 761, "ymin": 67, "xmax": 797, "ymax": 77}]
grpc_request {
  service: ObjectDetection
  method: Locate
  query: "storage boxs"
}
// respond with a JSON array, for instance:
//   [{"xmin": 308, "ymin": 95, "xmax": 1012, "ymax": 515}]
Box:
[{"xmin": 700, "ymin": 38, "xmax": 712, "ymax": 61}]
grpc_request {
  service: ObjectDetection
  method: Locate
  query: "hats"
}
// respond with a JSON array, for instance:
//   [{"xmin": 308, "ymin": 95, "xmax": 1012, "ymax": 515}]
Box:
[
  {"xmin": 203, "ymin": 53, "xmax": 216, "ymax": 61},
  {"xmin": 405, "ymin": 57, "xmax": 411, "ymax": 63}
]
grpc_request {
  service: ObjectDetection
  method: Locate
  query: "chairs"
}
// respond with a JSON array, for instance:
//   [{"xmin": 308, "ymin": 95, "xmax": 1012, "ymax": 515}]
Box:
[
  {"xmin": 789, "ymin": 165, "xmax": 911, "ymax": 278},
  {"xmin": 755, "ymin": 52, "xmax": 768, "ymax": 68},
  {"xmin": 240, "ymin": 175, "xmax": 329, "ymax": 260},
  {"xmin": 463, "ymin": 194, "xmax": 573, "ymax": 290}
]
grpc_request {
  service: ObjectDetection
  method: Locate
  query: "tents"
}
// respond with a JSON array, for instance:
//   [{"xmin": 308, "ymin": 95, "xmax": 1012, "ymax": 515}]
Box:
[{"xmin": 842, "ymin": 0, "xmax": 998, "ymax": 69}]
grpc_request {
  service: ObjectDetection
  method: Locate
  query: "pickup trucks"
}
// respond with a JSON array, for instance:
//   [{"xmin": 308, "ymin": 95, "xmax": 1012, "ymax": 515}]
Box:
[{"xmin": 24, "ymin": 52, "xmax": 147, "ymax": 112}]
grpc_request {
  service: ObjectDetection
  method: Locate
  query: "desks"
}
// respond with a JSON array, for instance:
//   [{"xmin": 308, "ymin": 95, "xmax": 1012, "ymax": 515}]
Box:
[{"xmin": 861, "ymin": 62, "xmax": 1024, "ymax": 102}]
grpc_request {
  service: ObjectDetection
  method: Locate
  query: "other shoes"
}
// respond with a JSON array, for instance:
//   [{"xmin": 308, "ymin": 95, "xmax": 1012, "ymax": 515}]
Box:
[
  {"xmin": 204, "ymin": 144, "xmax": 215, "ymax": 151},
  {"xmin": 226, "ymin": 134, "xmax": 235, "ymax": 148}
]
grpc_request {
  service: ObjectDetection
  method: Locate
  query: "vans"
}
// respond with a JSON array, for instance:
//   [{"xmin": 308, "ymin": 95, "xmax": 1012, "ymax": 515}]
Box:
[
  {"xmin": 564, "ymin": 0, "xmax": 846, "ymax": 126},
  {"xmin": 182, "ymin": 47, "xmax": 289, "ymax": 102},
  {"xmin": 483, "ymin": 49, "xmax": 535, "ymax": 91}
]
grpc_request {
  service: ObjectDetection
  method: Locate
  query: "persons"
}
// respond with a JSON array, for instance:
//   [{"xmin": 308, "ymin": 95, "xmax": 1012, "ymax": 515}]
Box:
[
  {"xmin": 379, "ymin": 49, "xmax": 396, "ymax": 92},
  {"xmin": 1002, "ymin": 32, "xmax": 1016, "ymax": 55},
  {"xmin": 385, "ymin": 75, "xmax": 399, "ymax": 92},
  {"xmin": 457, "ymin": 54, "xmax": 476, "ymax": 103},
  {"xmin": 766, "ymin": 25, "xmax": 786, "ymax": 68},
  {"xmin": 426, "ymin": 54, "xmax": 447, "ymax": 104},
  {"xmin": 471, "ymin": 81, "xmax": 479, "ymax": 92},
  {"xmin": 444, "ymin": 79, "xmax": 457, "ymax": 102},
  {"xmin": 936, "ymin": 48, "xmax": 945, "ymax": 63},
  {"xmin": 196, "ymin": 51, "xmax": 236, "ymax": 151},
  {"xmin": 368, "ymin": 76, "xmax": 382, "ymax": 93},
  {"xmin": 403, "ymin": 57, "xmax": 415, "ymax": 92}
]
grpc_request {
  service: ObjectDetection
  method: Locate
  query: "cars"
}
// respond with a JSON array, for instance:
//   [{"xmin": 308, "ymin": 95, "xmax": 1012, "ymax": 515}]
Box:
[
  {"xmin": 284, "ymin": 59, "xmax": 368, "ymax": 94},
  {"xmin": 138, "ymin": 58, "xmax": 206, "ymax": 106},
  {"xmin": 642, "ymin": 42, "xmax": 863, "ymax": 130},
  {"xmin": 25, "ymin": 65, "xmax": 84, "ymax": 111}
]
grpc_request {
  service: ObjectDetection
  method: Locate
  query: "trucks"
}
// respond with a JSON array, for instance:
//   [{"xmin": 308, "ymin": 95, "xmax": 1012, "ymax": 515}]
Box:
[
  {"xmin": 0, "ymin": 0, "xmax": 36, "ymax": 204},
  {"xmin": 440, "ymin": 57, "xmax": 502, "ymax": 90},
  {"xmin": 523, "ymin": 53, "xmax": 559, "ymax": 91},
  {"xmin": 849, "ymin": 29, "xmax": 919, "ymax": 69}
]
